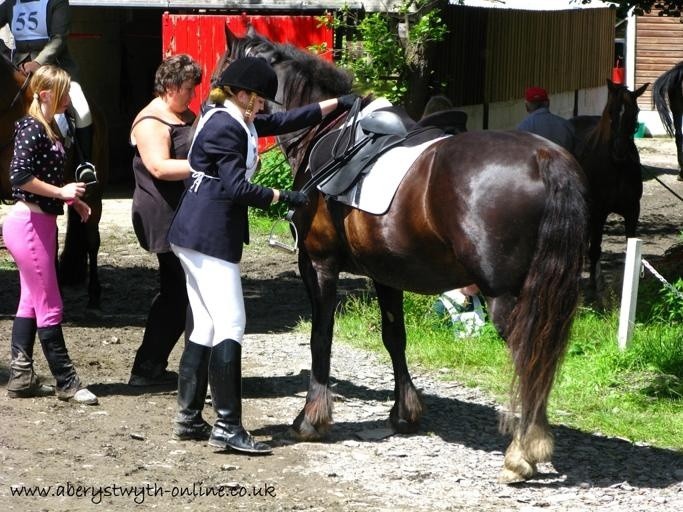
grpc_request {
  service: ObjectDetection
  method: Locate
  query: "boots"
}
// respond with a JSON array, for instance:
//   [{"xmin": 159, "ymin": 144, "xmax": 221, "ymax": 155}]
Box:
[
  {"xmin": 170, "ymin": 338, "xmax": 213, "ymax": 442},
  {"xmin": 74, "ymin": 123, "xmax": 96, "ymax": 183},
  {"xmin": 207, "ymin": 338, "xmax": 272, "ymax": 454},
  {"xmin": 37, "ymin": 322, "xmax": 99, "ymax": 404},
  {"xmin": 6, "ymin": 316, "xmax": 56, "ymax": 398}
]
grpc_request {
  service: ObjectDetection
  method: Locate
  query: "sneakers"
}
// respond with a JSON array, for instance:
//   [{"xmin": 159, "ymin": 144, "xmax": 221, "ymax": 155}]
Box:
[{"xmin": 129, "ymin": 369, "xmax": 178, "ymax": 387}]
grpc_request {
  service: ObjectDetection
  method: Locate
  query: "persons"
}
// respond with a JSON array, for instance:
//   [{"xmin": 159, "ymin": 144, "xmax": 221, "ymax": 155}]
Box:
[
  {"xmin": 1, "ymin": 1, "xmax": 94, "ymax": 164},
  {"xmin": 130, "ymin": 54, "xmax": 203, "ymax": 389},
  {"xmin": 3, "ymin": 66, "xmax": 101, "ymax": 405},
  {"xmin": 516, "ymin": 87, "xmax": 573, "ymax": 150},
  {"xmin": 164, "ymin": 57, "xmax": 366, "ymax": 454},
  {"xmin": 428, "ymin": 283, "xmax": 489, "ymax": 341}
]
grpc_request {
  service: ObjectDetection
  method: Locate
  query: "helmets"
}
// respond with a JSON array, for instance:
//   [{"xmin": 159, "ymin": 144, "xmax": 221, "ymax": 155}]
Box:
[{"xmin": 216, "ymin": 57, "xmax": 284, "ymax": 106}]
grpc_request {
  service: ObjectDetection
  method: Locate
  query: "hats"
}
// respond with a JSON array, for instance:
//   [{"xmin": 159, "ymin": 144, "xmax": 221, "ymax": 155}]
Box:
[{"xmin": 524, "ymin": 86, "xmax": 550, "ymax": 102}]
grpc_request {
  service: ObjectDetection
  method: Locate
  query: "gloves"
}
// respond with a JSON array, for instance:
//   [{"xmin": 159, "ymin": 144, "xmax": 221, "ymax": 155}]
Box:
[
  {"xmin": 335, "ymin": 92, "xmax": 362, "ymax": 110},
  {"xmin": 278, "ymin": 188, "xmax": 310, "ymax": 208}
]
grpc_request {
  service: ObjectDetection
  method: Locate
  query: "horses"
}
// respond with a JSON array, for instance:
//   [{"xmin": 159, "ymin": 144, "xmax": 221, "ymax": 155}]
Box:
[
  {"xmin": 568, "ymin": 79, "xmax": 652, "ymax": 291},
  {"xmin": 651, "ymin": 60, "xmax": 683, "ymax": 181},
  {"xmin": 185, "ymin": 21, "xmax": 593, "ymax": 484},
  {"xmin": 0, "ymin": 52, "xmax": 109, "ymax": 321}
]
